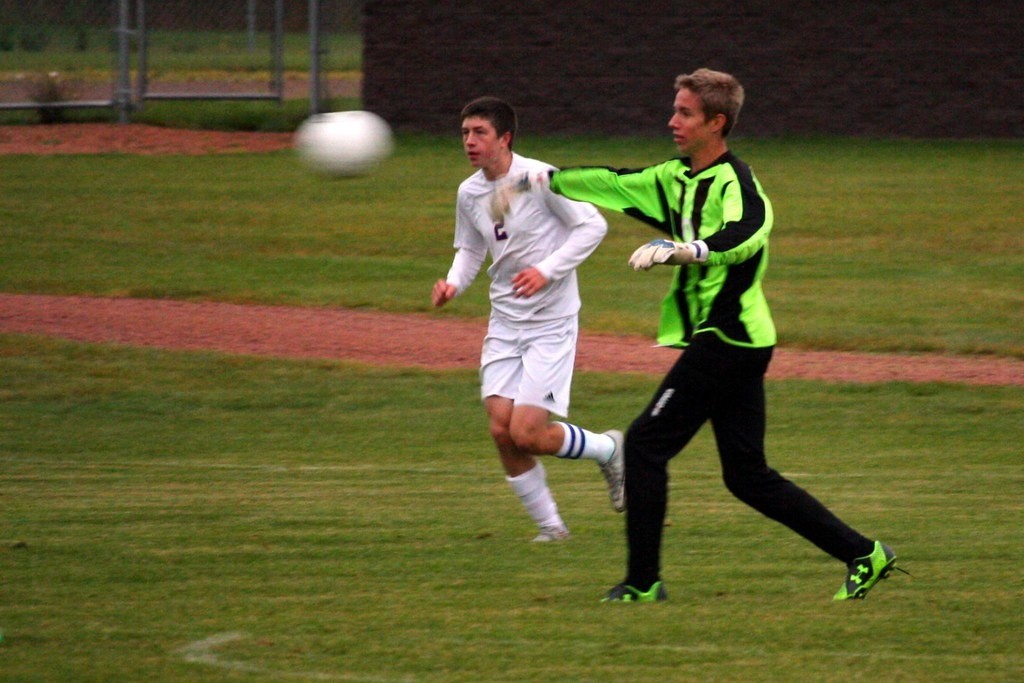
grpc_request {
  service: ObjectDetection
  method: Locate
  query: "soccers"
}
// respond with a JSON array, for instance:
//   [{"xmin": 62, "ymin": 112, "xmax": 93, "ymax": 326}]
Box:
[{"xmin": 291, "ymin": 109, "xmax": 394, "ymax": 176}]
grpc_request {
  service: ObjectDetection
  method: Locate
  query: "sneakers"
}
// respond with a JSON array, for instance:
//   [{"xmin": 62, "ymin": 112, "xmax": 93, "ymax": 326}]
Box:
[
  {"xmin": 533, "ymin": 527, "xmax": 567, "ymax": 544},
  {"xmin": 598, "ymin": 428, "xmax": 625, "ymax": 512},
  {"xmin": 602, "ymin": 579, "xmax": 669, "ymax": 607},
  {"xmin": 833, "ymin": 537, "xmax": 897, "ymax": 602}
]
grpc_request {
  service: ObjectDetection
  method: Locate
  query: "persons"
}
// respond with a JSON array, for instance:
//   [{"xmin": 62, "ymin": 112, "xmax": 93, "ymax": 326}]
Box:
[
  {"xmin": 429, "ymin": 94, "xmax": 625, "ymax": 541},
  {"xmin": 540, "ymin": 69, "xmax": 899, "ymax": 602}
]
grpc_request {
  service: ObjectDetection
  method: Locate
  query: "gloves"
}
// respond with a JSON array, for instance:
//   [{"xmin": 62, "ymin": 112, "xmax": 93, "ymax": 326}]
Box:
[{"xmin": 628, "ymin": 239, "xmax": 709, "ymax": 270}]
